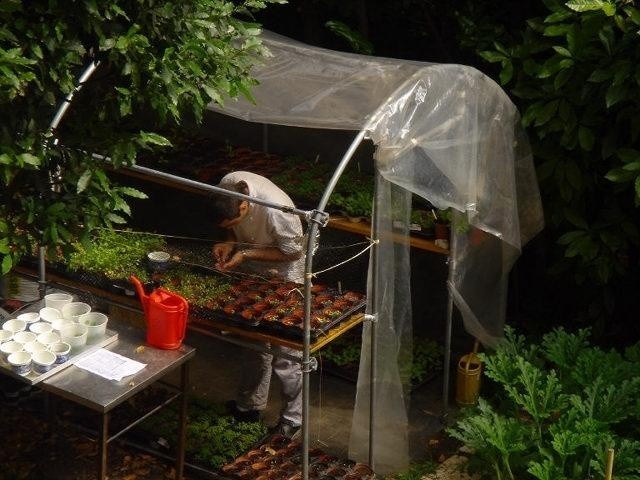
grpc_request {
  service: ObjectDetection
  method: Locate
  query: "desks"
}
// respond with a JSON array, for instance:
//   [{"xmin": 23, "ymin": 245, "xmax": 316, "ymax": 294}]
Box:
[{"xmin": 1, "ymin": 272, "xmax": 197, "ymax": 479}]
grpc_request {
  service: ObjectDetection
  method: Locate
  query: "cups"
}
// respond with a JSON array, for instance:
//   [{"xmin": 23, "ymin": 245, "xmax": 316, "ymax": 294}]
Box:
[
  {"xmin": 0, "ymin": 312, "xmax": 61, "ymax": 362},
  {"xmin": 50, "ymin": 341, "xmax": 72, "ymax": 366},
  {"xmin": 31, "ymin": 350, "xmax": 57, "ymax": 375},
  {"xmin": 39, "ymin": 306, "xmax": 61, "ymax": 323},
  {"xmin": 147, "ymin": 251, "xmax": 171, "ymax": 275},
  {"xmin": 7, "ymin": 351, "xmax": 31, "ymax": 377}
]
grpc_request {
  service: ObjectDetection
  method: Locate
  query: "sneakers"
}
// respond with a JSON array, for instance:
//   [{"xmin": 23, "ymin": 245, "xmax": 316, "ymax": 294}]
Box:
[
  {"xmin": 223, "ymin": 400, "xmax": 260, "ymax": 423},
  {"xmin": 270, "ymin": 416, "xmax": 303, "ymax": 440}
]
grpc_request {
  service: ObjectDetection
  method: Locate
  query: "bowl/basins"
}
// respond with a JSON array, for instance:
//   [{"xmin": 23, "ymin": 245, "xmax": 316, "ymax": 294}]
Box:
[
  {"xmin": 61, "ymin": 301, "xmax": 91, "ymax": 323},
  {"xmin": 79, "ymin": 312, "xmax": 109, "ymax": 339},
  {"xmin": 44, "ymin": 292, "xmax": 73, "ymax": 310},
  {"xmin": 61, "ymin": 324, "xmax": 89, "ymax": 352}
]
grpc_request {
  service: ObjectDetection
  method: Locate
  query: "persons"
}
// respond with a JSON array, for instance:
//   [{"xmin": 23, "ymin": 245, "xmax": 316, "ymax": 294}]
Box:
[
  {"xmin": 434, "ymin": 209, "xmax": 502, "ymax": 340},
  {"xmin": 206, "ymin": 171, "xmax": 306, "ymax": 438}
]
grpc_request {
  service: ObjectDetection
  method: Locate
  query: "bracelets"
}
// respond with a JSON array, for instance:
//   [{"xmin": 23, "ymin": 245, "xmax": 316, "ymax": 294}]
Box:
[{"xmin": 240, "ymin": 248, "xmax": 249, "ymax": 260}]
